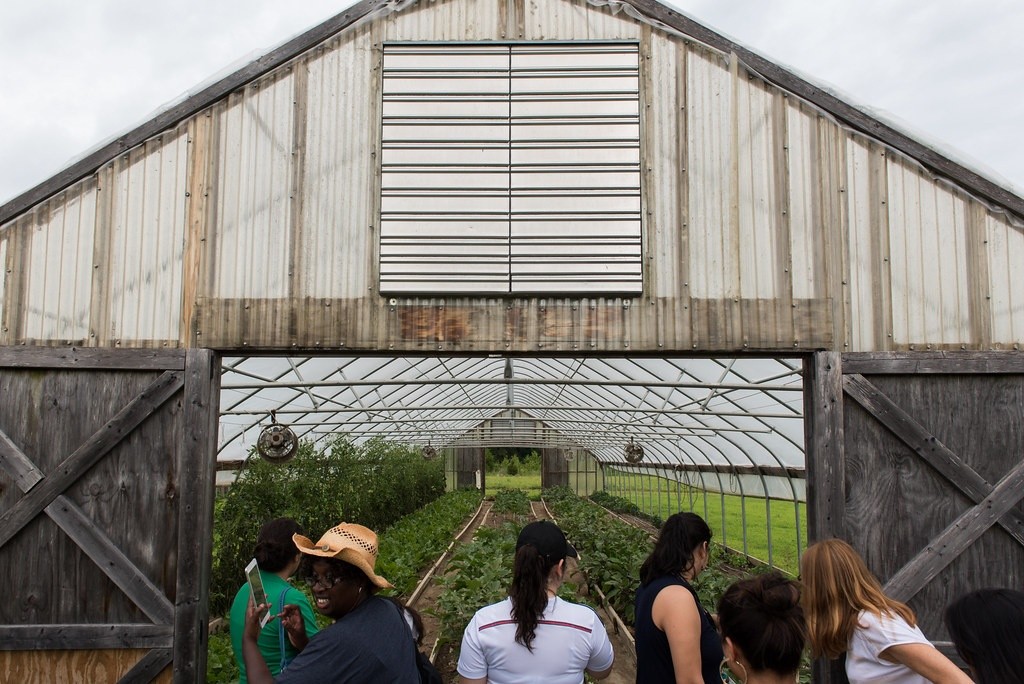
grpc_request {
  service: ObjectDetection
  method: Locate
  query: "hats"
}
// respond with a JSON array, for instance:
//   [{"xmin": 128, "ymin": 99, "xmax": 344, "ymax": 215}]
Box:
[
  {"xmin": 516, "ymin": 519, "xmax": 577, "ymax": 561},
  {"xmin": 292, "ymin": 521, "xmax": 396, "ymax": 588}
]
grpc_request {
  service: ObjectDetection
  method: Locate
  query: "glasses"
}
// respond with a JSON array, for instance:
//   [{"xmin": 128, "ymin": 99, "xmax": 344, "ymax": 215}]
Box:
[{"xmin": 304, "ymin": 576, "xmax": 344, "ymax": 589}]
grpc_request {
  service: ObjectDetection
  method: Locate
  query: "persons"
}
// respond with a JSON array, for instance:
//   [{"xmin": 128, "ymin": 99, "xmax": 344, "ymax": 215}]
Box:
[
  {"xmin": 635, "ymin": 511, "xmax": 724, "ymax": 684},
  {"xmin": 456, "ymin": 519, "xmax": 614, "ymax": 684},
  {"xmin": 716, "ymin": 573, "xmax": 807, "ymax": 684},
  {"xmin": 945, "ymin": 588, "xmax": 1024, "ymax": 684},
  {"xmin": 242, "ymin": 522, "xmax": 420, "ymax": 684},
  {"xmin": 230, "ymin": 518, "xmax": 320, "ymax": 684},
  {"xmin": 802, "ymin": 539, "xmax": 975, "ymax": 684}
]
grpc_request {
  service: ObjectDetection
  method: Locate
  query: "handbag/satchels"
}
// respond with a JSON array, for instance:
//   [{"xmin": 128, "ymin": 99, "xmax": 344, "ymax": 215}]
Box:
[{"xmin": 416, "ymin": 651, "xmax": 443, "ymax": 684}]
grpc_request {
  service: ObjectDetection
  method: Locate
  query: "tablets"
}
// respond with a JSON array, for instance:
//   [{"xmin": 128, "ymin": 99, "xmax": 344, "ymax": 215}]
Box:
[{"xmin": 245, "ymin": 558, "xmax": 271, "ymax": 629}]
